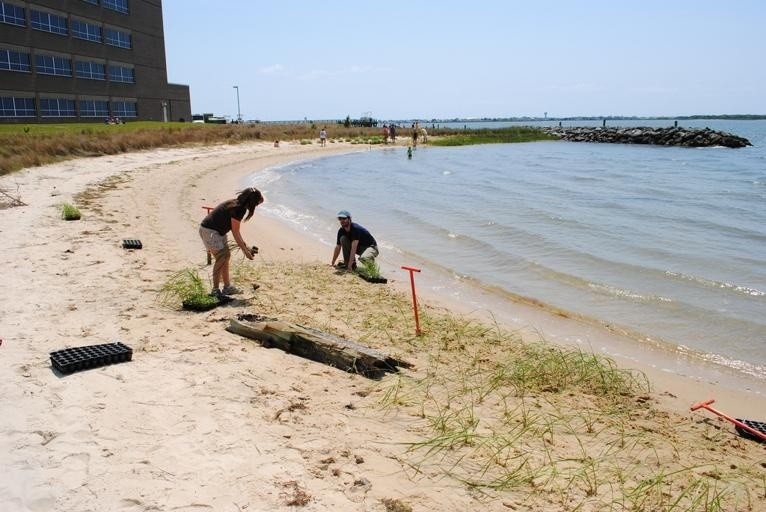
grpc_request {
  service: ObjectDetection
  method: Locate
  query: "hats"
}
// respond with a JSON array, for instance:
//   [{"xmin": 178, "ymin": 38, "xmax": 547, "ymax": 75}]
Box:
[{"xmin": 336, "ymin": 209, "xmax": 352, "ymax": 217}]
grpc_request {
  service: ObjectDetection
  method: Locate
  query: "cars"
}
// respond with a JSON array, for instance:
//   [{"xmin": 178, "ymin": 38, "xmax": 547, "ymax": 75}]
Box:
[{"xmin": 190, "ymin": 115, "xmax": 204, "ymax": 123}]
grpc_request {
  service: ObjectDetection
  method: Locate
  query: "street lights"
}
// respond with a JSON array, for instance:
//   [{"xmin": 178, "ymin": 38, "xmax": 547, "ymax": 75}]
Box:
[{"xmin": 232, "ymin": 86, "xmax": 240, "ymax": 120}]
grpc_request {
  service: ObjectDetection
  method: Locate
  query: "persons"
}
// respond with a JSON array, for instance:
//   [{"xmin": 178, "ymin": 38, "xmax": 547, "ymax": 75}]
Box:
[
  {"xmin": 326, "ymin": 209, "xmax": 379, "ymax": 277},
  {"xmin": 412, "ymin": 121, "xmax": 428, "ymax": 144},
  {"xmin": 383, "ymin": 122, "xmax": 396, "ymax": 144},
  {"xmin": 274, "ymin": 140, "xmax": 280, "ymax": 147},
  {"xmin": 407, "ymin": 146, "xmax": 413, "ymax": 157},
  {"xmin": 320, "ymin": 128, "xmax": 327, "ymax": 146},
  {"xmin": 199, "ymin": 187, "xmax": 263, "ymax": 299}
]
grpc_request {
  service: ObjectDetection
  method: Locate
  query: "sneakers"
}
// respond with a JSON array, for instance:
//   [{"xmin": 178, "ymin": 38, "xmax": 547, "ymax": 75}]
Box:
[
  {"xmin": 224, "ymin": 285, "xmax": 240, "ymax": 295},
  {"xmin": 211, "ymin": 288, "xmax": 222, "ymax": 295}
]
